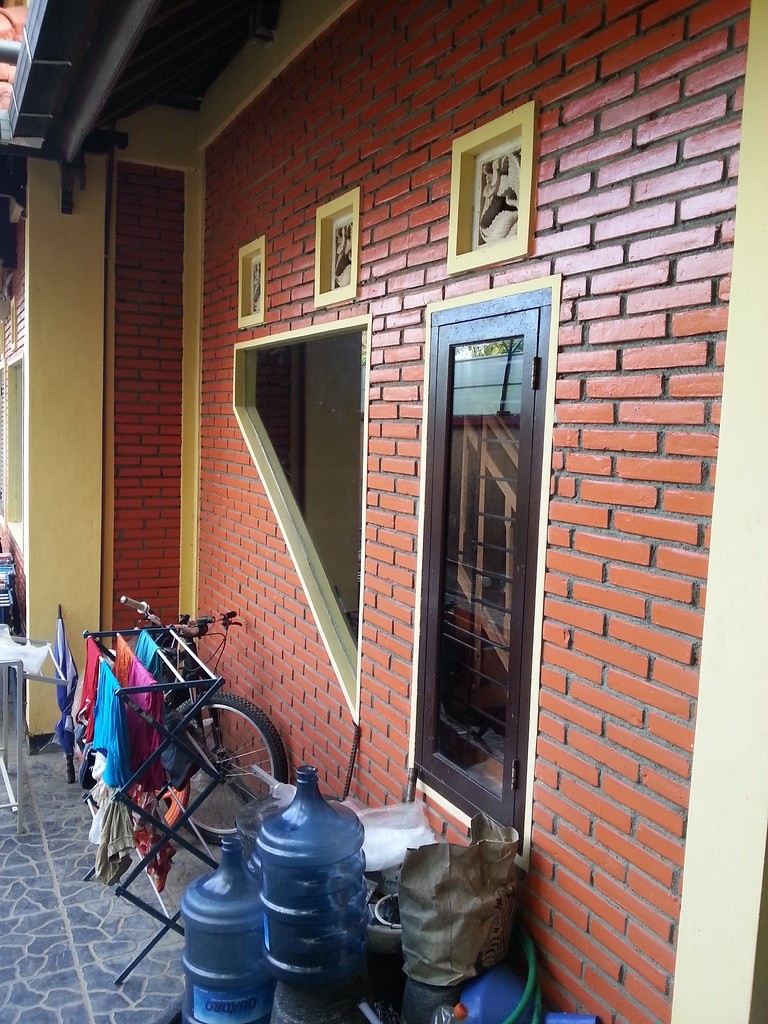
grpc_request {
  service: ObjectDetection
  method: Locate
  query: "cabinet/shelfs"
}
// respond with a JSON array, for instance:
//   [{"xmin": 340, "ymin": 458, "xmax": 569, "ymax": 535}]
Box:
[
  {"xmin": 82, "ymin": 627, "xmax": 226, "ymax": 986},
  {"xmin": 0, "ymin": 635, "xmax": 68, "ymax": 833}
]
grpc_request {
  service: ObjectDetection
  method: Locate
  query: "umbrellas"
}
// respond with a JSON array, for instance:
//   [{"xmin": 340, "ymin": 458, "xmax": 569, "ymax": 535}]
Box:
[{"xmin": 53, "ymin": 604, "xmax": 79, "ymax": 784}]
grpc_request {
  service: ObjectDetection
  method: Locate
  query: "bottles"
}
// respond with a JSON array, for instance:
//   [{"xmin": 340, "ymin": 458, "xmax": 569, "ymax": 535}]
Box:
[
  {"xmin": 256, "ymin": 765, "xmax": 370, "ymax": 986},
  {"xmin": 235, "ymin": 793, "xmax": 343, "ymax": 864},
  {"xmin": 430, "ymin": 1003, "xmax": 468, "ymax": 1024},
  {"xmin": 180, "ymin": 838, "xmax": 276, "ymax": 1024},
  {"xmin": 246, "ymin": 851, "xmax": 263, "ymax": 880}
]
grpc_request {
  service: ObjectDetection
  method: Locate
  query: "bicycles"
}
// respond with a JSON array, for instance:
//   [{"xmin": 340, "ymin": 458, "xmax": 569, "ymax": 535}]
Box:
[{"xmin": 98, "ymin": 595, "xmax": 289, "ymax": 847}]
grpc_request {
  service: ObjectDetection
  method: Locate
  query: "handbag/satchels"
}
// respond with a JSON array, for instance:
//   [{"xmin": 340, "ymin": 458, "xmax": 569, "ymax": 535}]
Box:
[{"xmin": 399, "ymin": 813, "xmax": 518, "ymax": 986}]
